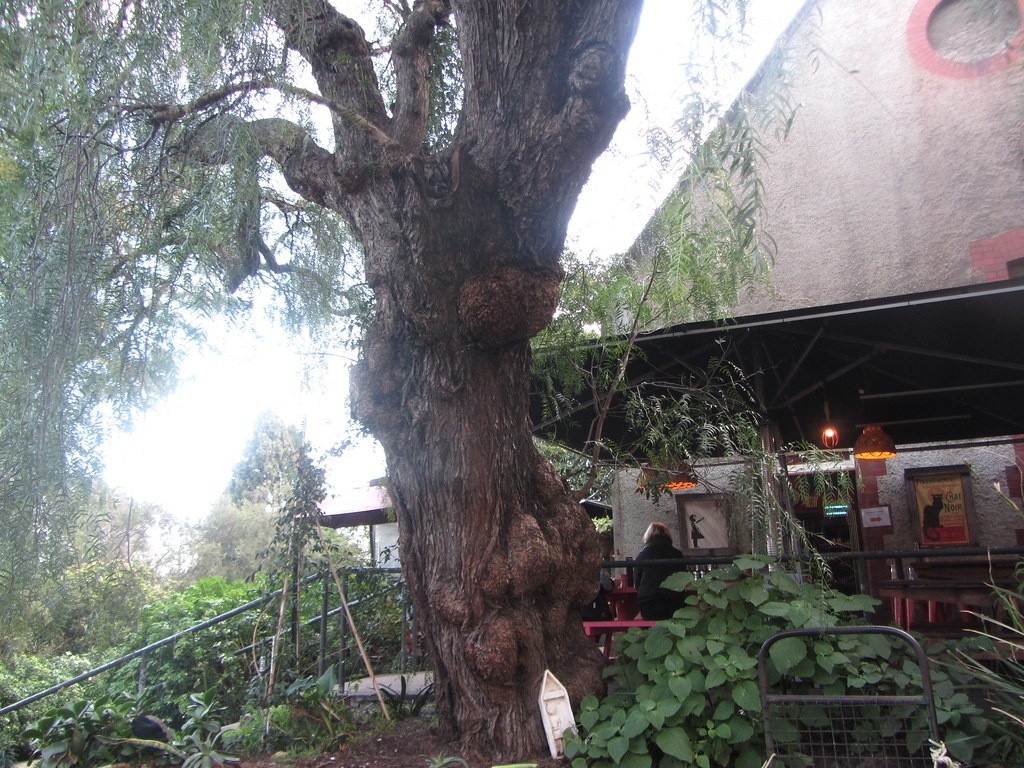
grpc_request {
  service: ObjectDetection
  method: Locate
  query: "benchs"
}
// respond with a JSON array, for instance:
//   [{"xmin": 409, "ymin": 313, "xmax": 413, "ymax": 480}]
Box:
[
  {"xmin": 583, "ymin": 620, "xmax": 673, "ymax": 635},
  {"xmin": 879, "ymin": 579, "xmax": 1019, "ymax": 631}
]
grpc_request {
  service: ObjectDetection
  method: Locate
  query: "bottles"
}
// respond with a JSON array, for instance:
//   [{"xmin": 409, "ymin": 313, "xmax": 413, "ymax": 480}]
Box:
[
  {"xmin": 891, "ymin": 563, "xmax": 898, "ymax": 580},
  {"xmin": 687, "ymin": 564, "xmax": 715, "ymax": 582},
  {"xmin": 909, "ymin": 567, "xmax": 915, "ymax": 580}
]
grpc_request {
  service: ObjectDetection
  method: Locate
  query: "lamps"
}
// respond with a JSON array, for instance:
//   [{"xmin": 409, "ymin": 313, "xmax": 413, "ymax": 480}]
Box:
[
  {"xmin": 635, "ymin": 455, "xmax": 699, "ymax": 490},
  {"xmin": 854, "ymin": 424, "xmax": 896, "ymax": 459},
  {"xmin": 820, "ymin": 400, "xmax": 838, "ymax": 449}
]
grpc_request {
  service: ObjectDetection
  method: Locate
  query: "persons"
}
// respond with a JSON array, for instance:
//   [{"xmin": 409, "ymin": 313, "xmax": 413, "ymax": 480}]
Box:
[
  {"xmin": 580, "ymin": 567, "xmax": 622, "ymax": 643},
  {"xmin": 634, "ymin": 522, "xmax": 688, "ymax": 621}
]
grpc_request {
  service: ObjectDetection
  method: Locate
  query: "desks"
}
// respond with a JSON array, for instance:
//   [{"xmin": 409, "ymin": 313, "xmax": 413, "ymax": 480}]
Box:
[
  {"xmin": 879, "ymin": 554, "xmax": 1022, "ymax": 630},
  {"xmin": 601, "ymin": 588, "xmax": 639, "ymax": 661}
]
